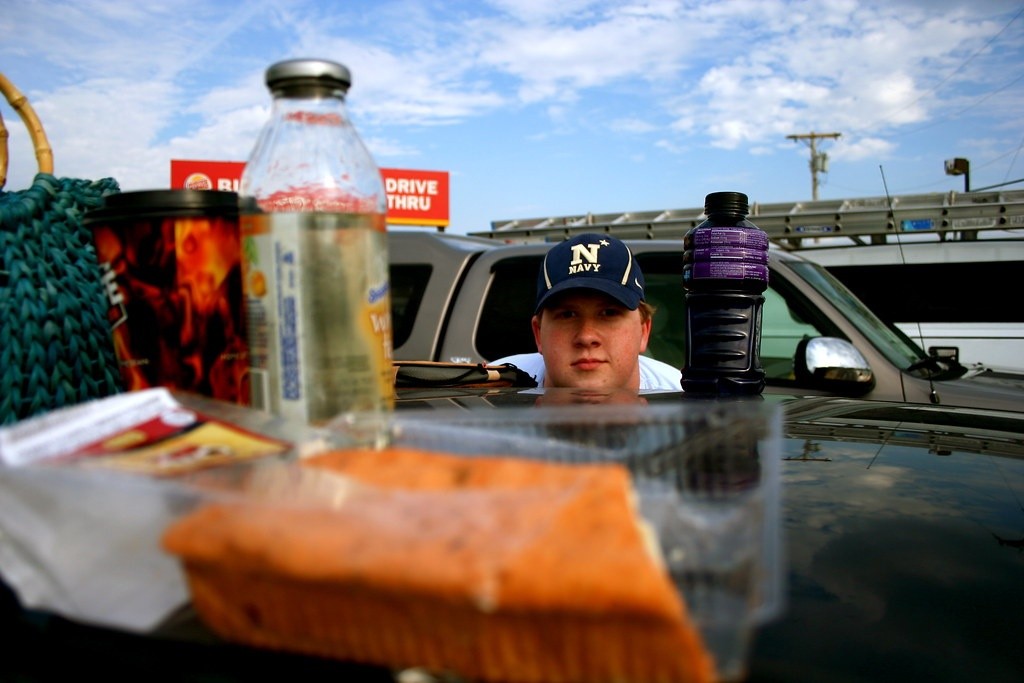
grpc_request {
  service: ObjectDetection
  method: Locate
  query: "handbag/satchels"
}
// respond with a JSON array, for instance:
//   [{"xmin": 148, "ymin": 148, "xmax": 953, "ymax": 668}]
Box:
[{"xmin": 1, "ymin": 72, "xmax": 132, "ymax": 430}]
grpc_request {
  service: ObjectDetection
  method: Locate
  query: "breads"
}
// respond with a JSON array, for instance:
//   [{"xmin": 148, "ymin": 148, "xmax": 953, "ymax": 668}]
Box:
[{"xmin": 162, "ymin": 448, "xmax": 720, "ymax": 683}]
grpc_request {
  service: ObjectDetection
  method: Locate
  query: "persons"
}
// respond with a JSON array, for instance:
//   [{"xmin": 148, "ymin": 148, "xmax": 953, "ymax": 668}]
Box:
[{"xmin": 483, "ymin": 234, "xmax": 682, "ymax": 392}]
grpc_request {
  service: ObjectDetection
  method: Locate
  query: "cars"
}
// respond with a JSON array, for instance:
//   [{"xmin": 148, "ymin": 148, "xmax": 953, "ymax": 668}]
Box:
[{"xmin": 0, "ymin": 386, "xmax": 1023, "ymax": 683}]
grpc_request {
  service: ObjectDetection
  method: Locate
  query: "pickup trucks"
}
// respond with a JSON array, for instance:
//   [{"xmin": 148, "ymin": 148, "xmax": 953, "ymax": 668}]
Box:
[{"xmin": 377, "ymin": 229, "xmax": 1024, "ymax": 412}]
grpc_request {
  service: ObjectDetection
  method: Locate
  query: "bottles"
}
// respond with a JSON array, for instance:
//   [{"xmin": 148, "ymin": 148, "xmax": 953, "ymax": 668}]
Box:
[
  {"xmin": 240, "ymin": 59, "xmax": 395, "ymax": 424},
  {"xmin": 680, "ymin": 192, "xmax": 769, "ymax": 399}
]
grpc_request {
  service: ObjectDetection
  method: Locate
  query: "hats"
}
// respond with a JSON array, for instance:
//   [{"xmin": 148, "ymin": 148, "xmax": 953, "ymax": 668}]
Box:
[{"xmin": 534, "ymin": 233, "xmax": 646, "ymax": 314}]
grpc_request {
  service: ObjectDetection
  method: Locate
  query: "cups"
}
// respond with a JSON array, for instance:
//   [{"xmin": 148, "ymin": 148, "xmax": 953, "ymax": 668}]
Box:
[{"xmin": 92, "ymin": 187, "xmax": 251, "ymax": 409}]
